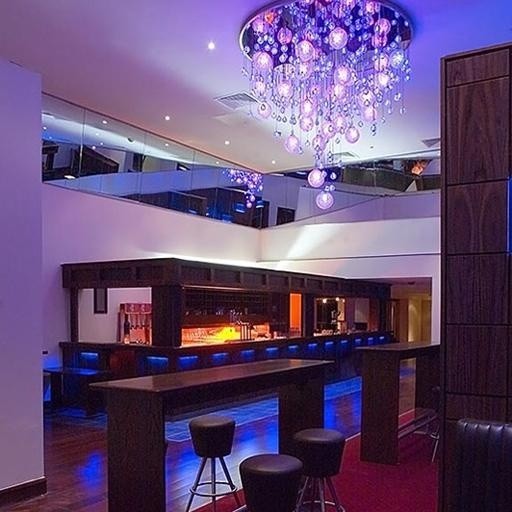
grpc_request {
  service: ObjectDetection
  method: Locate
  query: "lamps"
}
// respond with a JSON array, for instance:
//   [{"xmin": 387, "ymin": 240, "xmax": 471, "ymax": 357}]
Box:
[{"xmin": 226, "ymin": 0, "xmax": 413, "ymax": 210}]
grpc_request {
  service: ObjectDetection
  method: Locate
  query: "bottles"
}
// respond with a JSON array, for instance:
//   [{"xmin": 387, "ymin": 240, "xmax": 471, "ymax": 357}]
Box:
[
  {"xmin": 123, "ymin": 313, "xmax": 130, "ymax": 344},
  {"xmin": 240, "ymin": 323, "xmax": 250, "ymax": 339}
]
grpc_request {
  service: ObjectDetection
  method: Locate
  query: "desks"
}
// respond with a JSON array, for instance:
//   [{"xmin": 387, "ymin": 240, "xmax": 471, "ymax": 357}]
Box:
[
  {"xmin": 352, "ymin": 341, "xmax": 444, "ymax": 467},
  {"xmin": 88, "ymin": 353, "xmax": 337, "ymax": 512}
]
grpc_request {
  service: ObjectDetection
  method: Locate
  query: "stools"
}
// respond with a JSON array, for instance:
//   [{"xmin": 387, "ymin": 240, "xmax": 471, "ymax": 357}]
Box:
[
  {"xmin": 240, "ymin": 450, "xmax": 304, "ymax": 510},
  {"xmin": 290, "ymin": 427, "xmax": 348, "ymax": 512},
  {"xmin": 183, "ymin": 414, "xmax": 242, "ymax": 512}
]
